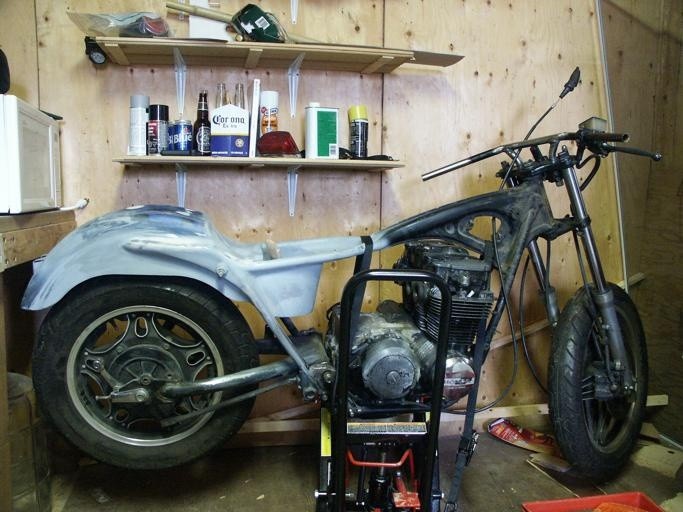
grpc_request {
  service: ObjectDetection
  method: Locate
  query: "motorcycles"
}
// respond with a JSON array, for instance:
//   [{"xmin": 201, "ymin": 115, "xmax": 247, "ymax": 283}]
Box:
[{"xmin": 12, "ymin": 69, "xmax": 660, "ymax": 482}]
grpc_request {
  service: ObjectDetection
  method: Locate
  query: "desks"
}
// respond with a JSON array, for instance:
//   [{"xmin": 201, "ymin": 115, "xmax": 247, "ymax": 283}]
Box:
[{"xmin": 0, "ymin": 211, "xmax": 76, "ymax": 512}]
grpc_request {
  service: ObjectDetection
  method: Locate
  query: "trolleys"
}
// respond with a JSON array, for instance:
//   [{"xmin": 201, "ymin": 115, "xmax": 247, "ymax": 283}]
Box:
[{"xmin": 317, "ymin": 267, "xmax": 449, "ymax": 512}]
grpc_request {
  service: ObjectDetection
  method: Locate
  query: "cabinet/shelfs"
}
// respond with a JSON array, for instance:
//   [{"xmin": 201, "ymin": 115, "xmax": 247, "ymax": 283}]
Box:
[{"xmin": 96, "ymin": 35, "xmax": 413, "ymax": 170}]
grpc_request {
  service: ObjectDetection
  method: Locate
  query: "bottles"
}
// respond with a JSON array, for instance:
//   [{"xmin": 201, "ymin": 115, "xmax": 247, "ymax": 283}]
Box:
[
  {"xmin": 346, "ymin": 105, "xmax": 368, "ymax": 159},
  {"xmin": 128, "ymin": 82, "xmax": 250, "ymax": 158},
  {"xmin": 258, "ymin": 91, "xmax": 279, "ymax": 157}
]
